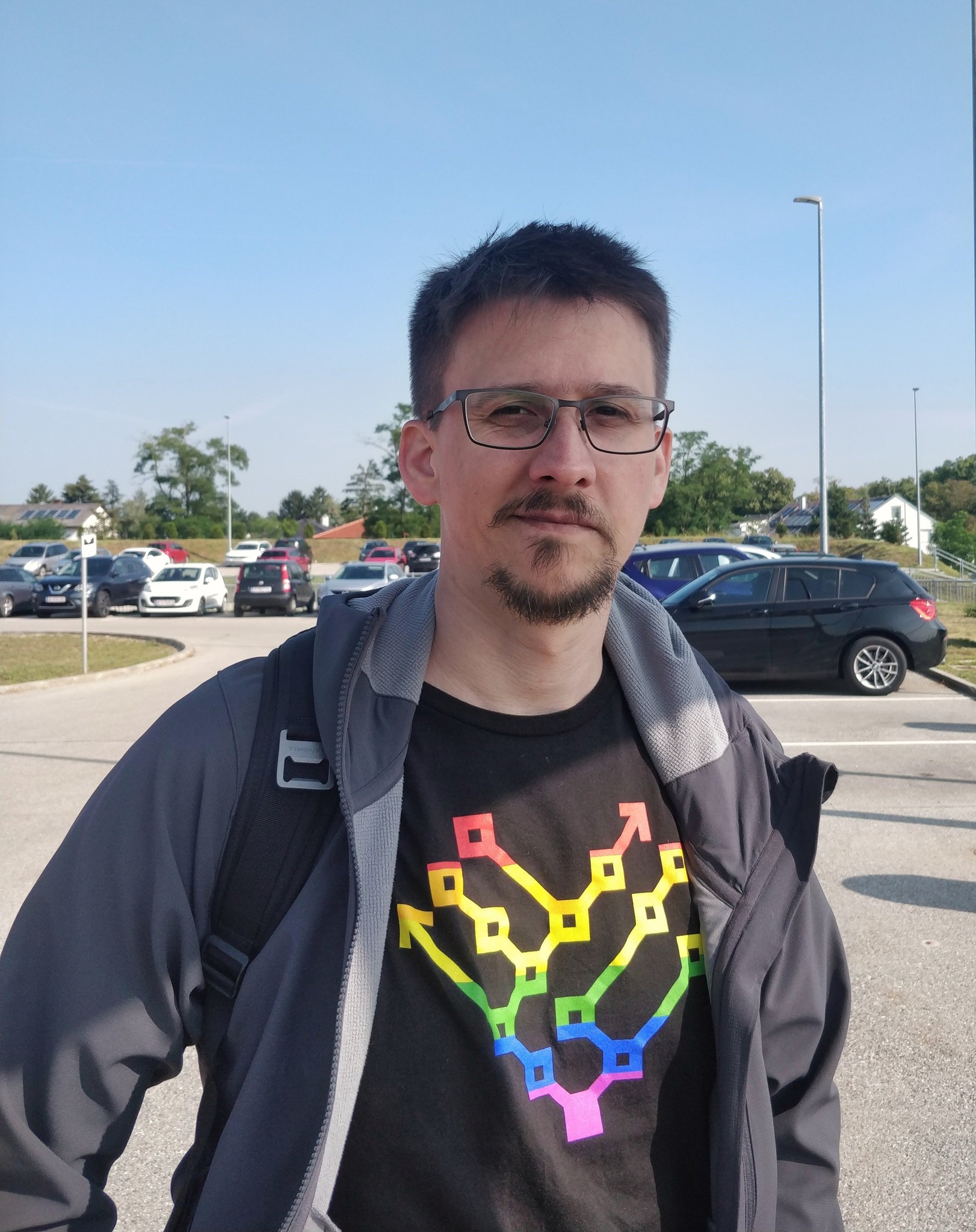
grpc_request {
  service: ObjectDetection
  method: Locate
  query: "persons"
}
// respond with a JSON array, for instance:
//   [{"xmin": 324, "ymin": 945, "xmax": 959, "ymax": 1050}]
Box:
[{"xmin": 0, "ymin": 222, "xmax": 852, "ymax": 1232}]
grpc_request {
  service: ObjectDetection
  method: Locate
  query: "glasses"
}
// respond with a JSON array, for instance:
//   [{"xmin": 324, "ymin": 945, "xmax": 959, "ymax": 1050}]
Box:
[{"xmin": 424, "ymin": 388, "xmax": 674, "ymax": 454}]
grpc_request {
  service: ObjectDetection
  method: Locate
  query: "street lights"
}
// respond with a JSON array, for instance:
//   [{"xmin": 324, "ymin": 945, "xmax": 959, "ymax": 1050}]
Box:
[
  {"xmin": 224, "ymin": 414, "xmax": 233, "ymax": 550},
  {"xmin": 913, "ymin": 385, "xmax": 922, "ymax": 567},
  {"xmin": 792, "ymin": 194, "xmax": 830, "ymax": 554}
]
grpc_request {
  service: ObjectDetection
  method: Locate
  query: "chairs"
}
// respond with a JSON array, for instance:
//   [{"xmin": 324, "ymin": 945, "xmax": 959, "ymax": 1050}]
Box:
[{"xmin": 669, "ymin": 558, "xmax": 680, "ymax": 578}]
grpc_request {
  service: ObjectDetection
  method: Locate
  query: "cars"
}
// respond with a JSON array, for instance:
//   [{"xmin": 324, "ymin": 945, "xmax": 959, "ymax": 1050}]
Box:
[
  {"xmin": 0, "ymin": 563, "xmax": 39, "ymax": 618},
  {"xmin": 633, "ymin": 535, "xmax": 799, "ymax": 556},
  {"xmin": 618, "ymin": 542, "xmax": 785, "ymax": 604},
  {"xmin": 233, "ymin": 560, "xmax": 315, "ymax": 617},
  {"xmin": 316, "ymin": 561, "xmax": 408, "ymax": 614},
  {"xmin": 3, "ymin": 541, "xmax": 70, "ymax": 577},
  {"xmin": 358, "ymin": 540, "xmax": 389, "ymax": 562},
  {"xmin": 51, "ymin": 545, "xmax": 112, "ymax": 575},
  {"xmin": 363, "ymin": 546, "xmax": 407, "ymax": 572},
  {"xmin": 112, "ymin": 547, "xmax": 171, "ymax": 574},
  {"xmin": 257, "ymin": 546, "xmax": 312, "ymax": 574},
  {"xmin": 223, "ymin": 540, "xmax": 274, "ymax": 567},
  {"xmin": 144, "ymin": 541, "xmax": 190, "ymax": 564},
  {"xmin": 138, "ymin": 563, "xmax": 229, "ymax": 616}
]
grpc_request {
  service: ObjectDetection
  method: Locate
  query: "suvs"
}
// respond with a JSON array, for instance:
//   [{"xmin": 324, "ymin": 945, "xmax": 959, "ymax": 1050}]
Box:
[
  {"xmin": 403, "ymin": 542, "xmax": 442, "ymax": 574},
  {"xmin": 272, "ymin": 537, "xmax": 314, "ymax": 563},
  {"xmin": 658, "ymin": 556, "xmax": 948, "ymax": 696},
  {"xmin": 31, "ymin": 553, "xmax": 155, "ymax": 618}
]
